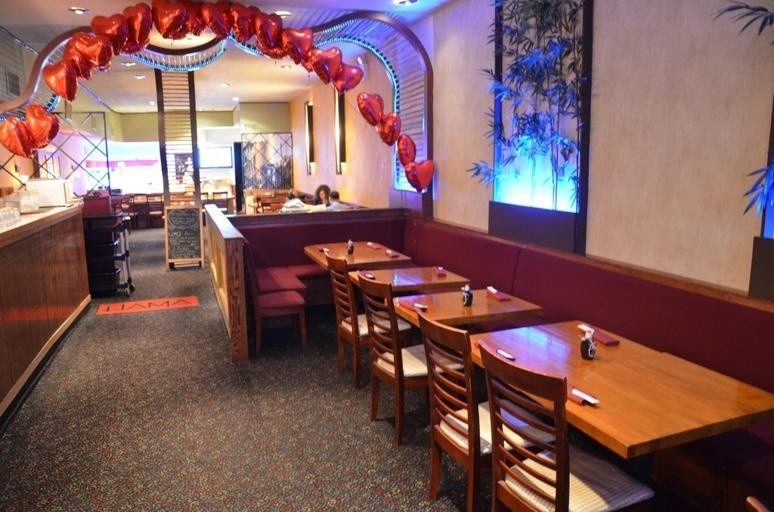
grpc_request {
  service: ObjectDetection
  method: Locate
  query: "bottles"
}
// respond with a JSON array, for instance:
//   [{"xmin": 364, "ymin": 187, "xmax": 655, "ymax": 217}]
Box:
[
  {"xmin": 461, "ymin": 284, "xmax": 473, "ymax": 307},
  {"xmin": 348, "ymin": 239, "xmax": 354, "ymax": 256},
  {"xmin": 580, "ymin": 329, "xmax": 597, "ymax": 360}
]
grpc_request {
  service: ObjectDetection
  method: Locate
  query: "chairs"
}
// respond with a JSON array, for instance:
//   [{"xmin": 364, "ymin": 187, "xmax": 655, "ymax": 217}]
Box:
[
  {"xmin": 415, "ymin": 311, "xmax": 558, "ymax": 511},
  {"xmin": 120, "ymin": 191, "xmax": 163, "ymax": 228},
  {"xmin": 478, "ymin": 344, "xmax": 656, "ymax": 511},
  {"xmin": 242, "ymin": 235, "xmax": 309, "ymax": 355},
  {"xmin": 325, "ymin": 256, "xmax": 413, "ymax": 389},
  {"xmin": 201, "ymin": 191, "xmax": 228, "ymax": 227},
  {"xmin": 357, "ymin": 272, "xmax": 467, "ymax": 445}
]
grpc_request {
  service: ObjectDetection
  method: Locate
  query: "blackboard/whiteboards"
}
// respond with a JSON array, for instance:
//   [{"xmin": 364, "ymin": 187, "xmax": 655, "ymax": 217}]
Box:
[{"xmin": 164, "ymin": 207, "xmax": 204, "ymax": 263}]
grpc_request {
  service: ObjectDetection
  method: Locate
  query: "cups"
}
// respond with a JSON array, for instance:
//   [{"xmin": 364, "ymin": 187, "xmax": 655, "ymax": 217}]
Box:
[{"xmin": 0, "ymin": 207, "xmax": 22, "ymax": 233}]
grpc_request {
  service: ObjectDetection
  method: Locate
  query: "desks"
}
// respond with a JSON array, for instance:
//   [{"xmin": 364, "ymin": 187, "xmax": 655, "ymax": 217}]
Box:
[
  {"xmin": 349, "ymin": 266, "xmax": 470, "ymax": 295},
  {"xmin": 300, "ymin": 241, "xmax": 413, "ymax": 280},
  {"xmin": 385, "ymin": 287, "xmax": 545, "ymax": 329}
]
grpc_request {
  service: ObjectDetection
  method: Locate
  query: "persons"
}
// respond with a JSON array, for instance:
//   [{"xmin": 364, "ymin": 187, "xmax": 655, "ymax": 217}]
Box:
[
  {"xmin": 279, "ymin": 189, "xmax": 311, "ymax": 213},
  {"xmin": 315, "ymin": 184, "xmax": 330, "ymax": 207},
  {"xmin": 326, "ymin": 191, "xmax": 346, "ymax": 208}
]
